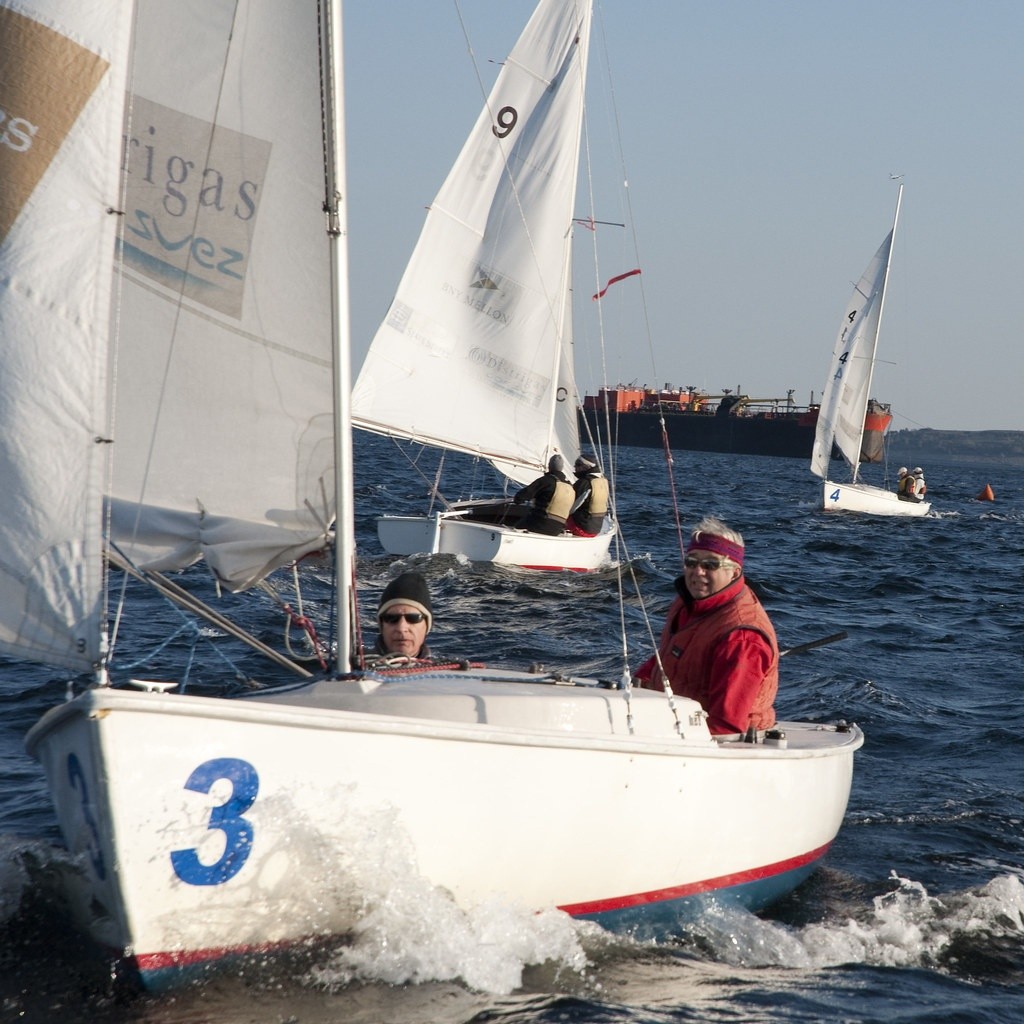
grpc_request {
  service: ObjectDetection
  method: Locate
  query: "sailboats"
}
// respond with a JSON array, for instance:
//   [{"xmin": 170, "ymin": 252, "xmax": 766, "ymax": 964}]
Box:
[
  {"xmin": 0, "ymin": 1, "xmax": 862, "ymax": 993},
  {"xmin": 343, "ymin": 1, "xmax": 621, "ymax": 572},
  {"xmin": 810, "ymin": 180, "xmax": 933, "ymax": 517}
]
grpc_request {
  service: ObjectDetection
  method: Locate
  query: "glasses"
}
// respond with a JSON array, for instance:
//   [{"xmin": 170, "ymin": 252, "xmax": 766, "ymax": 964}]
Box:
[
  {"xmin": 575, "ymin": 462, "xmax": 586, "ymax": 466},
  {"xmin": 684, "ymin": 556, "xmax": 720, "ymax": 572},
  {"xmin": 380, "ymin": 612, "xmax": 426, "ymax": 624}
]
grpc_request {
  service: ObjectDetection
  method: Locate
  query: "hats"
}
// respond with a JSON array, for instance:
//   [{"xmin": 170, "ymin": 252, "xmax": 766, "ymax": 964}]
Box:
[
  {"xmin": 376, "ymin": 572, "xmax": 433, "ymax": 636},
  {"xmin": 578, "ymin": 454, "xmax": 596, "ymax": 468}
]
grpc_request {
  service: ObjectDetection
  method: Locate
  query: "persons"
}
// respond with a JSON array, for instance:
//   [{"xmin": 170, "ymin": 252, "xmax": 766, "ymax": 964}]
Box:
[
  {"xmin": 566, "ymin": 453, "xmax": 610, "ymax": 537},
  {"xmin": 912, "ymin": 467, "xmax": 927, "ymax": 503},
  {"xmin": 631, "ymin": 519, "xmax": 779, "ymax": 735},
  {"xmin": 322, "ymin": 573, "xmax": 449, "ymax": 675},
  {"xmin": 897, "ymin": 467, "xmax": 915, "ymax": 502},
  {"xmin": 513, "ymin": 454, "xmax": 575, "ymax": 537}
]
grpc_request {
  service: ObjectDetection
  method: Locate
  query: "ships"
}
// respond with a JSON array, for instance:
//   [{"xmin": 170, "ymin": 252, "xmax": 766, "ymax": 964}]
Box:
[{"xmin": 577, "ymin": 377, "xmax": 893, "ymax": 464}]
